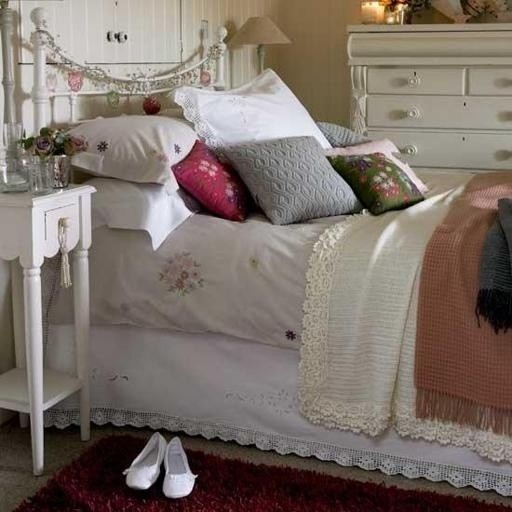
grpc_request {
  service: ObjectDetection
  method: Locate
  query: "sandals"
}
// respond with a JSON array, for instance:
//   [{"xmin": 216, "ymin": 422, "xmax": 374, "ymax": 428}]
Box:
[
  {"xmin": 162, "ymin": 437, "xmax": 199, "ymax": 498},
  {"xmin": 122, "ymin": 432, "xmax": 168, "ymax": 490}
]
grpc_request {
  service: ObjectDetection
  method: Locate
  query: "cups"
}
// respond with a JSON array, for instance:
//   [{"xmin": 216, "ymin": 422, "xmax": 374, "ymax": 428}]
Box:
[
  {"xmin": 360, "ymin": 2, "xmax": 385, "ymax": 25},
  {"xmin": 385, "ymin": 12, "xmax": 396, "ymax": 25},
  {"xmin": 48, "ymin": 156, "xmax": 71, "ymax": 189},
  {"xmin": 26, "ymin": 163, "xmax": 54, "ymax": 196}
]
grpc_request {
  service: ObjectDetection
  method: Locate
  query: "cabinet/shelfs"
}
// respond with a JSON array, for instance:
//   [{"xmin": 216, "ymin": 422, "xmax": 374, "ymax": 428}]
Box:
[{"xmin": 345, "ymin": 23, "xmax": 512, "ymax": 188}]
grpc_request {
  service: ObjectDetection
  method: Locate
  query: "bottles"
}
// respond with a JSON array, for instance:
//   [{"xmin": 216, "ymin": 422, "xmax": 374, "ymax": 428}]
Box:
[
  {"xmin": 399, "ymin": 10, "xmax": 406, "ymax": 25},
  {"xmin": 0, "ymin": 123, "xmax": 35, "ymax": 193}
]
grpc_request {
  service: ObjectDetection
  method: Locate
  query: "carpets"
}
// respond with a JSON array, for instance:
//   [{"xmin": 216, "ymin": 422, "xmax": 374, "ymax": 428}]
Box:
[{"xmin": 18, "ymin": 434, "xmax": 512, "ymax": 512}]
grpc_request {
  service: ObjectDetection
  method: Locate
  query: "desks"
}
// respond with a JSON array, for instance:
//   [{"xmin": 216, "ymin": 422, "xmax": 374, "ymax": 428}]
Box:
[{"xmin": 0, "ymin": 186, "xmax": 95, "ymax": 476}]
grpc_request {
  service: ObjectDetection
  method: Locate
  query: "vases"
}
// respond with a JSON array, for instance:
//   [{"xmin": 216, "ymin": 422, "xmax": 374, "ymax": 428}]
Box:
[{"xmin": 34, "ymin": 155, "xmax": 67, "ymax": 187}]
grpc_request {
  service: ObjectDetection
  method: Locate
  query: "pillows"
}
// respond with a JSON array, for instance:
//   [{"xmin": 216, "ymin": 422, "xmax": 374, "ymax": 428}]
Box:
[
  {"xmin": 329, "ymin": 138, "xmax": 429, "ymax": 193},
  {"xmin": 316, "ymin": 121, "xmax": 371, "ymax": 149},
  {"xmin": 326, "ymin": 152, "xmax": 425, "ymax": 214},
  {"xmin": 171, "ymin": 139, "xmax": 254, "ymax": 222},
  {"xmin": 224, "ymin": 136, "xmax": 363, "ymax": 228},
  {"xmin": 167, "ymin": 68, "xmax": 334, "ymax": 162},
  {"xmin": 64, "ymin": 116, "xmax": 197, "ymax": 185},
  {"xmin": 79, "ymin": 178, "xmax": 204, "ymax": 252}
]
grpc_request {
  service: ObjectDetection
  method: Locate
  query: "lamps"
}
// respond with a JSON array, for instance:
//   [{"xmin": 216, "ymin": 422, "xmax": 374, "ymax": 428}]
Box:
[{"xmin": 228, "ymin": 16, "xmax": 292, "ymax": 74}]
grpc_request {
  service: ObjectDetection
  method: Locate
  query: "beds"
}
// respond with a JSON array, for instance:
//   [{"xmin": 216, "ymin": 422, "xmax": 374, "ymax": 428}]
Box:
[{"xmin": 28, "ymin": 6, "xmax": 512, "ymax": 496}]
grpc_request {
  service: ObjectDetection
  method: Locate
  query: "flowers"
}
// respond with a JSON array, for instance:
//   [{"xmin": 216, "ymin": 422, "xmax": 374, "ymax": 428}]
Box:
[{"xmin": 18, "ymin": 127, "xmax": 89, "ymax": 161}]
